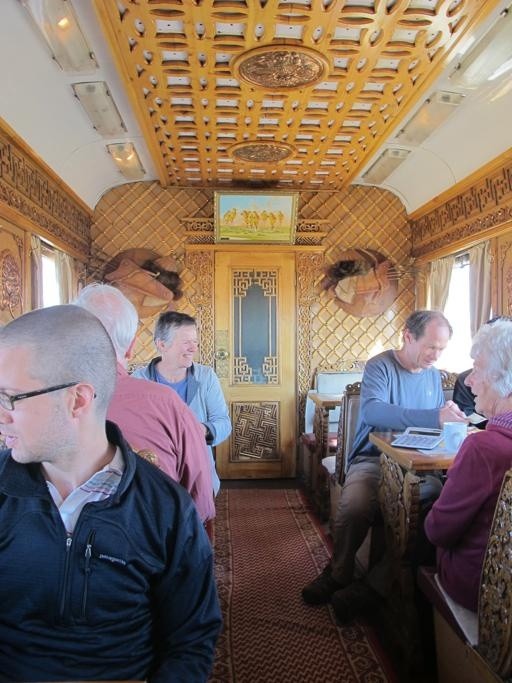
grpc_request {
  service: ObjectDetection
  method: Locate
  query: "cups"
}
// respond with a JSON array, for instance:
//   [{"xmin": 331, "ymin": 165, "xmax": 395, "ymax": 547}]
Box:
[{"xmin": 441, "ymin": 420, "xmax": 468, "ymax": 453}]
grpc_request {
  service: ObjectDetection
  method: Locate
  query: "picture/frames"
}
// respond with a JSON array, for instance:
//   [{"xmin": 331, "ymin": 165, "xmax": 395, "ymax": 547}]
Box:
[{"xmin": 213, "ymin": 189, "xmax": 299, "ymax": 245}]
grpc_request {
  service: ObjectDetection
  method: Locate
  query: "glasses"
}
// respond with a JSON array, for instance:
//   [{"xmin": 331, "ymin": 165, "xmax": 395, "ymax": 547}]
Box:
[{"xmin": 0, "ymin": 382, "xmax": 97, "ymax": 411}]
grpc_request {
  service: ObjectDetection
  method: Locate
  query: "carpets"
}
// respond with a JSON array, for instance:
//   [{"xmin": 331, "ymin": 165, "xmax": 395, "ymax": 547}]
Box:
[{"xmin": 204, "ymin": 487, "xmax": 396, "ymax": 681}]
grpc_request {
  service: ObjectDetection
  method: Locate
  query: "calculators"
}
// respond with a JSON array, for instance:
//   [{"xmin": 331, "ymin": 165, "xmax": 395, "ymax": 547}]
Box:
[{"xmin": 390, "ymin": 426, "xmax": 445, "ymax": 450}]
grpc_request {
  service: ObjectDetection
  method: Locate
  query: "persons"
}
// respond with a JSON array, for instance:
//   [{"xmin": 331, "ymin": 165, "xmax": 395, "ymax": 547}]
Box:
[
  {"xmin": 127, "ymin": 307, "xmax": 234, "ymax": 502},
  {"xmin": 1, "ymin": 301, "xmax": 226, "ymax": 682},
  {"xmin": 293, "ymin": 306, "xmax": 470, "ymax": 609},
  {"xmin": 448, "ymin": 314, "xmax": 512, "ymax": 428},
  {"xmin": 67, "ymin": 279, "xmax": 218, "ymax": 548},
  {"xmin": 420, "ymin": 316, "xmax": 512, "ymax": 612}
]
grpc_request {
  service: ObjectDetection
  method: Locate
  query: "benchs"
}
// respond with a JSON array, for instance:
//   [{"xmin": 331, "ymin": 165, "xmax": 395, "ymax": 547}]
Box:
[{"xmin": 298, "ymin": 369, "xmax": 512, "ymax": 682}]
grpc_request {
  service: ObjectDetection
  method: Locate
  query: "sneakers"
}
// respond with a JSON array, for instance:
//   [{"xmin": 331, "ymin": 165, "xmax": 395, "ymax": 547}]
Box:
[{"xmin": 304, "ymin": 565, "xmax": 353, "ymax": 605}]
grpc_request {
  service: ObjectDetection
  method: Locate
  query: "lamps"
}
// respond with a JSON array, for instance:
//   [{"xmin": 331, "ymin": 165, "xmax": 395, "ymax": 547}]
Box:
[
  {"xmin": 19, "ymin": 0, "xmax": 145, "ymax": 181},
  {"xmin": 361, "ymin": 0, "xmax": 511, "ymax": 186}
]
